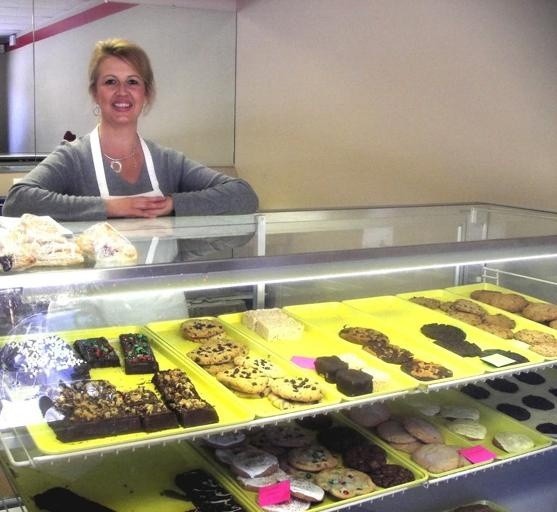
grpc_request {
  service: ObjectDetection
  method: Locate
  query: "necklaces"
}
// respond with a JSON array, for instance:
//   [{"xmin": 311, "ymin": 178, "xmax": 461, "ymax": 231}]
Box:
[{"xmin": 99, "ymin": 142, "xmax": 143, "ymax": 174}]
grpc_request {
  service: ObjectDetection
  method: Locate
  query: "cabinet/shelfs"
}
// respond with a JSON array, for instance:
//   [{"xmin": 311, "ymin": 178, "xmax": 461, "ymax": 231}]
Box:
[{"xmin": 1, "ymin": 199, "xmax": 557, "ymax": 512}]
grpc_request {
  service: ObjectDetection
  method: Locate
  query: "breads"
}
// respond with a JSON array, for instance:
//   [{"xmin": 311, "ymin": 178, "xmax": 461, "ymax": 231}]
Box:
[{"xmin": 0, "ymin": 213, "xmax": 138, "ymax": 272}]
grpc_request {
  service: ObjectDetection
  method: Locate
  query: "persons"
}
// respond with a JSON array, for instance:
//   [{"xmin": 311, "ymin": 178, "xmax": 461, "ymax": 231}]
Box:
[{"xmin": 0, "ymin": 37, "xmax": 260, "ymax": 330}]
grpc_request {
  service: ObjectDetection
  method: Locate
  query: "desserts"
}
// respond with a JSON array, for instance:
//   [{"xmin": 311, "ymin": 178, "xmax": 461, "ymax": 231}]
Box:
[
  {"xmin": 1, "ymin": 290, "xmax": 247, "ymax": 512},
  {"xmin": 181, "ymin": 290, "xmax": 557, "ymax": 512}
]
